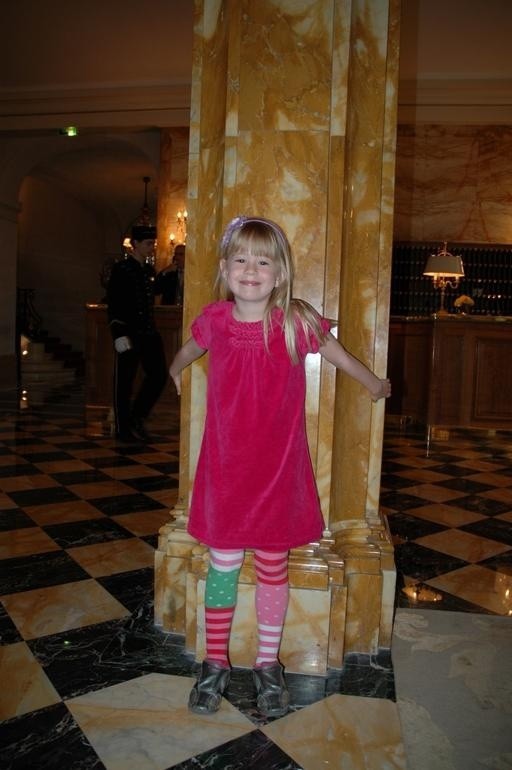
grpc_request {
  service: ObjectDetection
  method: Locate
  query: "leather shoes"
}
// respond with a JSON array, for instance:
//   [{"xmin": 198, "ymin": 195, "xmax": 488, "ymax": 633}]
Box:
[
  {"xmin": 113, "ymin": 423, "xmax": 151, "ymax": 445},
  {"xmin": 186, "ymin": 655, "xmax": 234, "ymax": 719},
  {"xmin": 247, "ymin": 659, "xmax": 297, "ymax": 722}
]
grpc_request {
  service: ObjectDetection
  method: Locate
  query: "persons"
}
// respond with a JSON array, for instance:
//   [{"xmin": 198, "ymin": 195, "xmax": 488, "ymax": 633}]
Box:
[
  {"xmin": 105, "ymin": 224, "xmax": 171, "ymax": 445},
  {"xmin": 166, "ymin": 215, "xmax": 393, "ymax": 719},
  {"xmin": 154, "ymin": 244, "xmax": 185, "ymax": 306}
]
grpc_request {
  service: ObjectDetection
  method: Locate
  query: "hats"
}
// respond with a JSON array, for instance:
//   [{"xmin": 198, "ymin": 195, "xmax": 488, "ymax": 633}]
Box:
[{"xmin": 130, "ymin": 223, "xmax": 158, "ymax": 243}]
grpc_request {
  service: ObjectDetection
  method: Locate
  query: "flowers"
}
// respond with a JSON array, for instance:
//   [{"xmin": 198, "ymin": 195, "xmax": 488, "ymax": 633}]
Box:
[{"xmin": 454, "ymin": 294, "xmax": 475, "ymax": 307}]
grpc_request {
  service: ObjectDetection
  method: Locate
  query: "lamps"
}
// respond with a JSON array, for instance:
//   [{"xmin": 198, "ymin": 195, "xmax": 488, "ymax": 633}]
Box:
[
  {"xmin": 121, "ymin": 177, "xmax": 157, "ymax": 259},
  {"xmin": 57, "ymin": 127, "xmax": 78, "ymax": 136},
  {"xmin": 170, "ymin": 210, "xmax": 187, "ymax": 248},
  {"xmin": 422, "ymin": 241, "xmax": 466, "ymax": 316}
]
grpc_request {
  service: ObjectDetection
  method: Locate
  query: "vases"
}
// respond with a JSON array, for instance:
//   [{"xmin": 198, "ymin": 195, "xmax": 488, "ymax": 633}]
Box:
[{"xmin": 461, "ymin": 307, "xmax": 469, "ymax": 313}]
